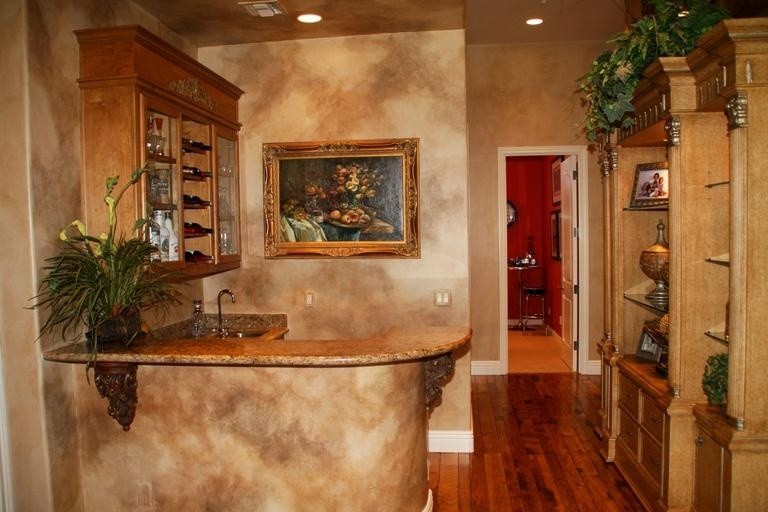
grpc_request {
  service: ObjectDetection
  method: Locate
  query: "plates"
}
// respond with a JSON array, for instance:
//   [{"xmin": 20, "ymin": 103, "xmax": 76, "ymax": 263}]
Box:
[{"xmin": 330, "ymin": 219, "xmax": 367, "ymax": 229}]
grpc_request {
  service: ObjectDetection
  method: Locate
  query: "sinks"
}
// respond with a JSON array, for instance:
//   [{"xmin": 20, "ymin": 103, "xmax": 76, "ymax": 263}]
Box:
[{"xmin": 213, "ymin": 328, "xmax": 270, "ymax": 339}]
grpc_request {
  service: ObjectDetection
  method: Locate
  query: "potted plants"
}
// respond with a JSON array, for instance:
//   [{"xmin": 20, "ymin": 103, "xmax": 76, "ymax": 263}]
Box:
[{"xmin": 23, "ymin": 162, "xmax": 191, "ymax": 386}]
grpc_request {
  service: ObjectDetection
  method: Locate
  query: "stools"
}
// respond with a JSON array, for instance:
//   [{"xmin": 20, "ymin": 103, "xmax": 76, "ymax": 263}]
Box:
[{"xmin": 522, "ymin": 285, "xmax": 547, "ymax": 336}]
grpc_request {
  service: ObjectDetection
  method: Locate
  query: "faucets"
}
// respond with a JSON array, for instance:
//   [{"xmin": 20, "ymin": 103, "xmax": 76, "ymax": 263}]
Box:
[{"xmin": 217, "ymin": 288, "xmax": 237, "ymax": 330}]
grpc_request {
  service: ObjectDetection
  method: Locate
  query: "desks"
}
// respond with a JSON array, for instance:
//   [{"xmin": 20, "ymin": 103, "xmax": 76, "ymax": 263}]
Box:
[{"xmin": 508, "ymin": 264, "xmax": 542, "ymax": 331}]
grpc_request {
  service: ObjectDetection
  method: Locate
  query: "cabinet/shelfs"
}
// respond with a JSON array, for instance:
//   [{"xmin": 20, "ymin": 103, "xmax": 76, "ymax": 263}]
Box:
[
  {"xmin": 621, "ymin": 128, "xmax": 670, "ymax": 384},
  {"xmin": 613, "ymin": 368, "xmax": 665, "ymax": 512},
  {"xmin": 696, "ymin": 111, "xmax": 731, "ymax": 417},
  {"xmin": 74, "ymin": 23, "xmax": 244, "ymax": 280},
  {"xmin": 695, "ymin": 429, "xmax": 724, "ymax": 512}
]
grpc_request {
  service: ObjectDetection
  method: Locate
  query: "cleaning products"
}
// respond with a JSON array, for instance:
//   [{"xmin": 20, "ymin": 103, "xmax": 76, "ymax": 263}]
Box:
[{"xmin": 191, "ymin": 299, "xmax": 207, "ymax": 337}]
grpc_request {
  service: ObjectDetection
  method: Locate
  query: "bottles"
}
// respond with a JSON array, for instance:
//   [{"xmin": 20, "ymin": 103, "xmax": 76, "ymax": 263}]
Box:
[
  {"xmin": 189, "ymin": 300, "xmax": 208, "ymax": 343},
  {"xmin": 183, "ymin": 138, "xmax": 214, "ymax": 263},
  {"xmin": 514, "ymin": 253, "xmax": 529, "ymax": 264},
  {"xmin": 147, "ymin": 114, "xmax": 179, "ymax": 262}
]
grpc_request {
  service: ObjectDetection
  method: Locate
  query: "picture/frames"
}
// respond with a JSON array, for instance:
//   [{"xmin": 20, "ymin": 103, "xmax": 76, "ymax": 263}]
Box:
[
  {"xmin": 550, "ymin": 210, "xmax": 561, "ymax": 259},
  {"xmin": 261, "ymin": 136, "xmax": 422, "ymax": 262},
  {"xmin": 630, "ymin": 161, "xmax": 669, "ymax": 207},
  {"xmin": 551, "ymin": 155, "xmax": 565, "ymax": 205},
  {"xmin": 637, "ymin": 329, "xmax": 662, "ymax": 365}
]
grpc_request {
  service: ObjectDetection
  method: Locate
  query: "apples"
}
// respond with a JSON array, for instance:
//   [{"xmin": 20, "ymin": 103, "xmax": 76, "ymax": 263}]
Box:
[{"xmin": 308, "ymin": 182, "xmax": 370, "ymax": 224}]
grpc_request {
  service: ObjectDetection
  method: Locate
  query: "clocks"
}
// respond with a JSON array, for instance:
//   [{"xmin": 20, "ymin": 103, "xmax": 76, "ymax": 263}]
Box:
[{"xmin": 507, "ymin": 200, "xmax": 517, "ymax": 226}]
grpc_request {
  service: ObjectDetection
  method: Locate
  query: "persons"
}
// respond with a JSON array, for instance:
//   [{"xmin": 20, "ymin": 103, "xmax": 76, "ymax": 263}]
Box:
[
  {"xmin": 638, "ymin": 173, "xmax": 666, "ymax": 197},
  {"xmin": 644, "ymin": 335, "xmax": 655, "ymax": 353}
]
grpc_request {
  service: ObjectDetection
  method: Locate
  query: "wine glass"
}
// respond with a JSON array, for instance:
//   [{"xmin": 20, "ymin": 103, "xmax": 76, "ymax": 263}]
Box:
[{"xmin": 219, "ymin": 139, "xmax": 236, "ymax": 254}]
